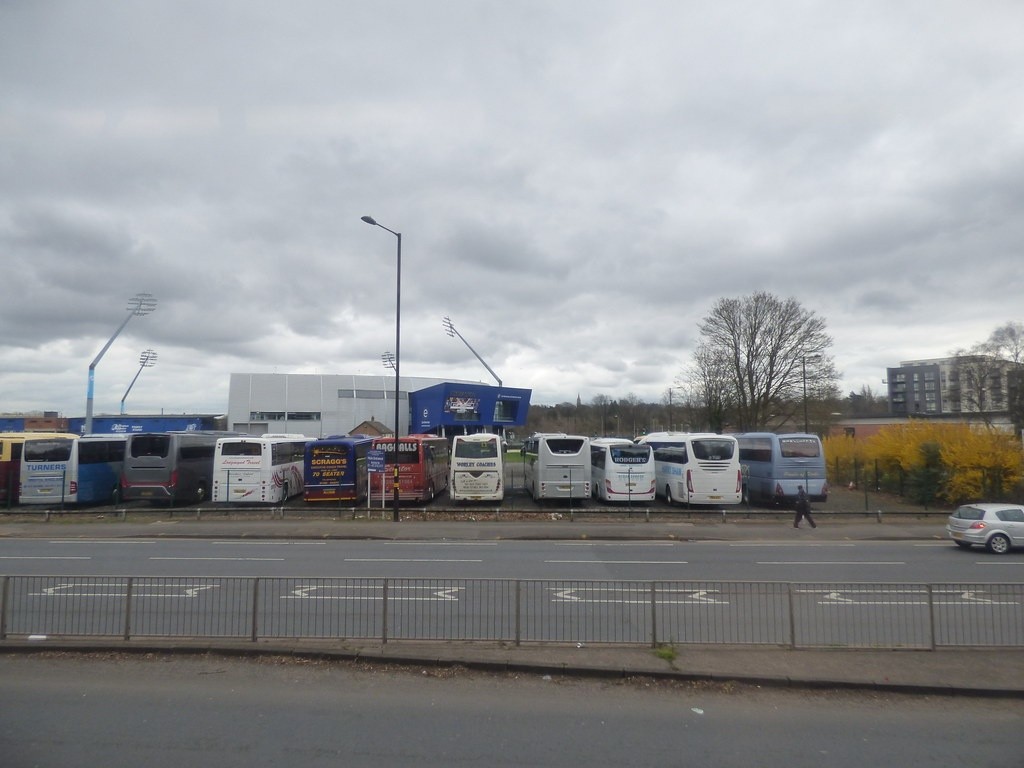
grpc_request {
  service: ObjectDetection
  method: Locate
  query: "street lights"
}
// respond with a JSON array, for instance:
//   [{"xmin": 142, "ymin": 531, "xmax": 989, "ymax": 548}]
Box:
[
  {"xmin": 361, "ymin": 216, "xmax": 403, "ymax": 521},
  {"xmin": 802, "ymin": 354, "xmax": 822, "ymax": 434}
]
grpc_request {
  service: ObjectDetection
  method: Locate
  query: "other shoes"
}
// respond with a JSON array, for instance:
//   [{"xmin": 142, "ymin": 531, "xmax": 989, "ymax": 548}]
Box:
[
  {"xmin": 809, "ymin": 526, "xmax": 818, "ymax": 529},
  {"xmin": 791, "ymin": 527, "xmax": 799, "ymax": 529}
]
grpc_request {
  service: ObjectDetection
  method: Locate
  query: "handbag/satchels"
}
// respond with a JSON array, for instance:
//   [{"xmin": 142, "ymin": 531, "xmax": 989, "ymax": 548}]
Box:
[{"xmin": 793, "ymin": 501, "xmax": 811, "ymax": 512}]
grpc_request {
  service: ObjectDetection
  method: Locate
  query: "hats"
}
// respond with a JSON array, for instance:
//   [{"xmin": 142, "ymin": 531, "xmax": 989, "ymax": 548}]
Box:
[{"xmin": 798, "ymin": 486, "xmax": 802, "ymax": 489}]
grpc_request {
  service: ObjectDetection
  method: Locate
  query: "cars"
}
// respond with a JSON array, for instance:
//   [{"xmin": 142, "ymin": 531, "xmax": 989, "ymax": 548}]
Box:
[{"xmin": 946, "ymin": 501, "xmax": 1024, "ymax": 554}]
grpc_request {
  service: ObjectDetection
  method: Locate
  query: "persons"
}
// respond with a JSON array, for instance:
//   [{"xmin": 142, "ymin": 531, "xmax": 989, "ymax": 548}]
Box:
[{"xmin": 791, "ymin": 485, "xmax": 817, "ymax": 529}]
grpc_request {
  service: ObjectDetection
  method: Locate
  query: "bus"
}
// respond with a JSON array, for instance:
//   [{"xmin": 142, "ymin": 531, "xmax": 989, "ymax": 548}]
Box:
[
  {"xmin": 119, "ymin": 427, "xmax": 244, "ymax": 507},
  {"xmin": 720, "ymin": 430, "xmax": 829, "ymax": 506},
  {"xmin": 588, "ymin": 437, "xmax": 658, "ymax": 504},
  {"xmin": 19, "ymin": 434, "xmax": 130, "ymax": 509},
  {"xmin": 449, "ymin": 431, "xmax": 508, "ymax": 503},
  {"xmin": 631, "ymin": 430, "xmax": 744, "ymax": 507},
  {"xmin": 212, "ymin": 433, "xmax": 318, "ymax": 505},
  {"xmin": 0, "ymin": 433, "xmax": 80, "ymax": 508},
  {"xmin": 301, "ymin": 433, "xmax": 378, "ymax": 508},
  {"xmin": 367, "ymin": 433, "xmax": 451, "ymax": 507},
  {"xmin": 520, "ymin": 431, "xmax": 592, "ymax": 505}
]
